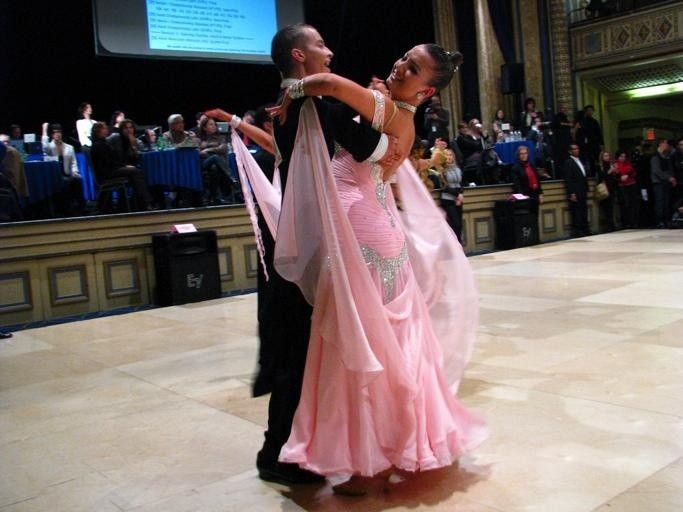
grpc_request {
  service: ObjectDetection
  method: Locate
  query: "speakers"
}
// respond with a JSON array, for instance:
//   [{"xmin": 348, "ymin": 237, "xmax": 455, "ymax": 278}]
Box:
[{"xmin": 501, "ymin": 61, "xmax": 524, "ymax": 95}]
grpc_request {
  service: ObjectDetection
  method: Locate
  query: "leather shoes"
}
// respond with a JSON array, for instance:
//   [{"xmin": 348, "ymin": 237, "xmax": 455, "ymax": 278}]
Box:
[{"xmin": 255, "ymin": 452, "xmax": 325, "ymax": 483}]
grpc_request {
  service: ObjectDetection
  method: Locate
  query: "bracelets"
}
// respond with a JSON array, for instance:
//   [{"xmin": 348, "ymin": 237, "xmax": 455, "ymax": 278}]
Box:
[{"xmin": 286, "ymin": 78, "xmax": 305, "ymax": 100}]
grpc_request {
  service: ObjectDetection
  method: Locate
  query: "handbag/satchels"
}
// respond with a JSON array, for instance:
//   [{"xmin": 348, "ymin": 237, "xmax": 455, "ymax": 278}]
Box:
[{"xmin": 594, "ymin": 184, "xmax": 609, "ymax": 202}]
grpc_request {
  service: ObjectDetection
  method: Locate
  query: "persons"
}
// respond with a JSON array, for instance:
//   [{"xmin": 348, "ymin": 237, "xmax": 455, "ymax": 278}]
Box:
[
  {"xmin": 253, "ymin": 23, "xmax": 404, "ymax": 490},
  {"xmin": 264, "ymin": 44, "xmax": 491, "ymax": 486},
  {"xmin": 407, "ymin": 96, "xmax": 604, "ymax": 248},
  {"xmin": 8, "ymin": 102, "xmax": 278, "ymax": 213},
  {"xmin": 596, "ymin": 136, "xmax": 681, "ymax": 233}
]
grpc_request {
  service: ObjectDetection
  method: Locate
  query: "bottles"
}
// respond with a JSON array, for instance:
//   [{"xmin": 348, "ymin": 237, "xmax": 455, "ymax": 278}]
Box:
[
  {"xmin": 144, "ymin": 133, "xmax": 193, "ymax": 153},
  {"xmin": 494, "ymin": 128, "xmax": 523, "ymax": 145}
]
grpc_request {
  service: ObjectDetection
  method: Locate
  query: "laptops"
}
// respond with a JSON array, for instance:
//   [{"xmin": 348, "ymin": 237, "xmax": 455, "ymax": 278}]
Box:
[{"xmin": 214, "ymin": 122, "xmax": 230, "ymax": 133}]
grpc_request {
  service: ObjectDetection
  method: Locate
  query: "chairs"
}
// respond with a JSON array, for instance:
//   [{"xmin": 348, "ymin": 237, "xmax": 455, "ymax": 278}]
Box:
[
  {"xmin": 1, "ymin": 131, "xmax": 255, "ymax": 213},
  {"xmin": 419, "ymin": 131, "xmax": 567, "ymax": 190}
]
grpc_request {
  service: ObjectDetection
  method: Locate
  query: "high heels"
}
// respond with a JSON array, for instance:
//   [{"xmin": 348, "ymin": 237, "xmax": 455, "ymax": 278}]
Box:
[{"xmin": 333, "ymin": 466, "xmax": 395, "ymax": 496}]
